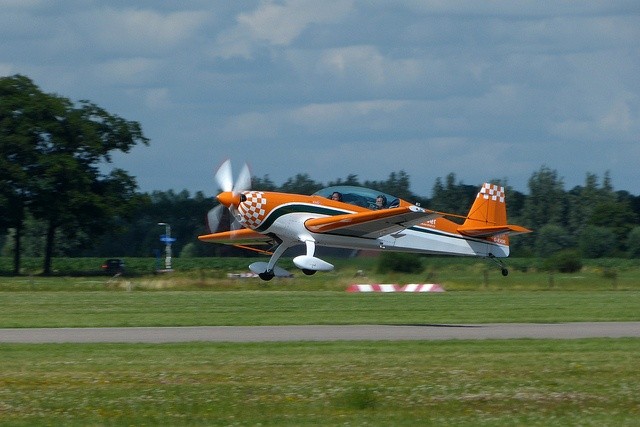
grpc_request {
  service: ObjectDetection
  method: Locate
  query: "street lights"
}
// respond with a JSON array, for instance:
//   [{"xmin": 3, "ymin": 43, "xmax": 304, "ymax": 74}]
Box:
[{"xmin": 157, "ymin": 223, "xmax": 176, "ymax": 269}]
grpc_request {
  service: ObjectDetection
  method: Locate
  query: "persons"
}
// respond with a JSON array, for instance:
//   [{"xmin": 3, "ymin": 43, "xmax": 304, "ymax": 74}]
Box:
[
  {"xmin": 375, "ymin": 195, "xmax": 387, "ymax": 209},
  {"xmin": 331, "ymin": 192, "xmax": 342, "ymax": 200}
]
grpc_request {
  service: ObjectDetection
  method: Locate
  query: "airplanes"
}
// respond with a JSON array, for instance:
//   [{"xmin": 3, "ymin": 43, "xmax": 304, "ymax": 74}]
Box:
[{"xmin": 198, "ymin": 159, "xmax": 534, "ymax": 281}]
